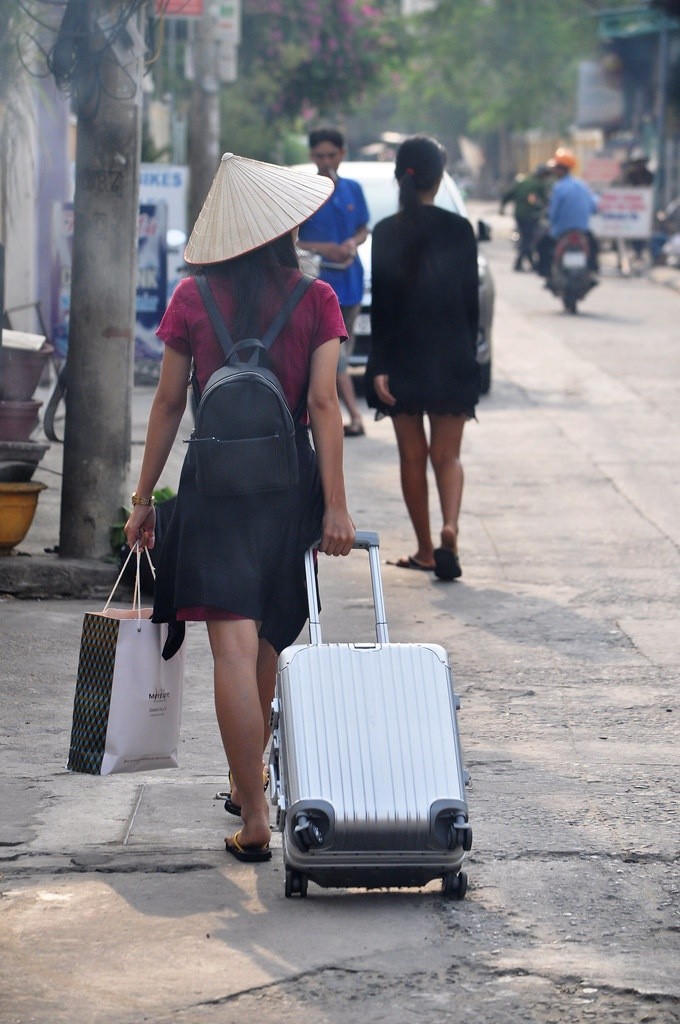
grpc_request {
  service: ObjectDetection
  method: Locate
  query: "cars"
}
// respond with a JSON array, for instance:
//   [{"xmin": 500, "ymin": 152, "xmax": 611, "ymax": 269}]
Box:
[{"xmin": 286, "ymin": 161, "xmax": 494, "ymax": 393}]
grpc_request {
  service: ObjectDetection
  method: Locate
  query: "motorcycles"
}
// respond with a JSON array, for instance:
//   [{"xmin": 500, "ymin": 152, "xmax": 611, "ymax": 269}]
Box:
[{"xmin": 527, "ymin": 193, "xmax": 598, "ymax": 312}]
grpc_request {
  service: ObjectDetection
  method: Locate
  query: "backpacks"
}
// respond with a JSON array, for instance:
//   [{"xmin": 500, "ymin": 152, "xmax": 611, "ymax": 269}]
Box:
[{"xmin": 184, "ymin": 275, "xmax": 317, "ymax": 491}]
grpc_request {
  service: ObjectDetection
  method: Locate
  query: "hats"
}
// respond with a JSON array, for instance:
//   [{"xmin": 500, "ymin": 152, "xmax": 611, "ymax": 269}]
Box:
[{"xmin": 183, "ymin": 151, "xmax": 335, "ymax": 266}]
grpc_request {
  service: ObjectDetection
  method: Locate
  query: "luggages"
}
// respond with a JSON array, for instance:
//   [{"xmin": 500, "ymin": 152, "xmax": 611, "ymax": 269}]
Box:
[{"xmin": 269, "ymin": 528, "xmax": 473, "ymax": 901}]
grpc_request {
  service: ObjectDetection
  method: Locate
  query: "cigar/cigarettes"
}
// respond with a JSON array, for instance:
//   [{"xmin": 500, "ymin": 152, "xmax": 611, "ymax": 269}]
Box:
[{"xmin": 328, "ymin": 167, "xmax": 338, "ymax": 182}]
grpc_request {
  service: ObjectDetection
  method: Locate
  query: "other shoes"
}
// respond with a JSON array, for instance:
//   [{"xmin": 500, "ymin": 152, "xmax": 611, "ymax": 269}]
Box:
[{"xmin": 344, "ymin": 423, "xmax": 365, "ymax": 437}]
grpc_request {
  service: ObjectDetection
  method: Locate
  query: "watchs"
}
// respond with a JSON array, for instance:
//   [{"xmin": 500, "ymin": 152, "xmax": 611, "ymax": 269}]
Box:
[{"xmin": 131, "ymin": 492, "xmax": 155, "ymax": 507}]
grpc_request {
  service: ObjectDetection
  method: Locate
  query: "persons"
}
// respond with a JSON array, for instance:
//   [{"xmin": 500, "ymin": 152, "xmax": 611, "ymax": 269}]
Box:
[
  {"xmin": 498, "ymin": 146, "xmax": 652, "ymax": 291},
  {"xmin": 362, "ymin": 136, "xmax": 482, "ymax": 581},
  {"xmin": 125, "ymin": 153, "xmax": 354, "ymax": 862},
  {"xmin": 294, "ymin": 128, "xmax": 369, "ymax": 439}
]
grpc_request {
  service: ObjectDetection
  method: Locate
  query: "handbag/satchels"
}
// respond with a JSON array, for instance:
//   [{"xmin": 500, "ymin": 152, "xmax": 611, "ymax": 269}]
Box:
[{"xmin": 64, "ymin": 539, "xmax": 185, "ymax": 777}]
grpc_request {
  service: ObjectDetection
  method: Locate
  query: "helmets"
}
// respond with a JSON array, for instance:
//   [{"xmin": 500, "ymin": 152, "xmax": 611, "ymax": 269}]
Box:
[
  {"xmin": 629, "ymin": 146, "xmax": 650, "ymax": 164},
  {"xmin": 554, "ymin": 147, "xmax": 576, "ymax": 169}
]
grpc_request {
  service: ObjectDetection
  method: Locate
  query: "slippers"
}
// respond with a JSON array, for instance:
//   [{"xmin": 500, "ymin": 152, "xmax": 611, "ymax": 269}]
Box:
[
  {"xmin": 225, "ymin": 829, "xmax": 273, "ymax": 863},
  {"xmin": 386, "ymin": 556, "xmax": 434, "ymax": 571},
  {"xmin": 223, "ymin": 770, "xmax": 270, "ymax": 816},
  {"xmin": 432, "ymin": 549, "xmax": 462, "ymax": 580}
]
